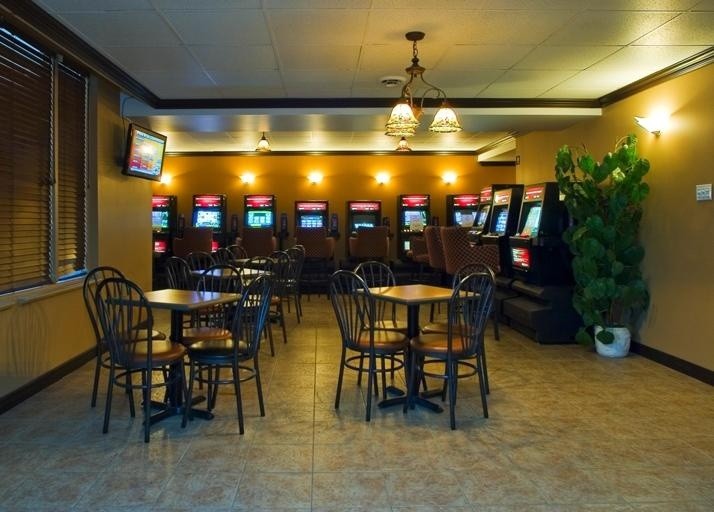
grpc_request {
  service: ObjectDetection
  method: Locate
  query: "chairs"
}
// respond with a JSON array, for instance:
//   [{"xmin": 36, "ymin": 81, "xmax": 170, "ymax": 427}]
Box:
[
  {"xmin": 424, "ymin": 225, "xmax": 444, "ymax": 269},
  {"xmin": 172, "ymin": 228, "xmax": 213, "ymax": 259},
  {"xmin": 410, "ymin": 224, "xmax": 429, "ymax": 264},
  {"xmin": 235, "ymin": 227, "xmax": 277, "ymax": 258},
  {"xmin": 440, "ymin": 225, "xmax": 501, "ymax": 276},
  {"xmin": 348, "ymin": 225, "xmax": 390, "ymax": 263},
  {"xmin": 297, "ymin": 225, "xmax": 336, "ymax": 258}
]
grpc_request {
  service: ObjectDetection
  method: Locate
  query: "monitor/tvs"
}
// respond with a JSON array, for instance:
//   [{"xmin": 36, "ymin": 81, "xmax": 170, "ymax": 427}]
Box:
[
  {"xmin": 245, "ymin": 209, "xmax": 274, "ymax": 229},
  {"xmin": 353, "ymin": 213, "xmax": 376, "ymax": 229},
  {"xmin": 151, "ymin": 210, "xmax": 169, "ymax": 229},
  {"xmin": 300, "ymin": 213, "xmax": 324, "ymax": 228},
  {"xmin": 122, "ymin": 123, "xmax": 167, "ymax": 182},
  {"xmin": 454, "ymin": 205, "xmax": 541, "ymax": 237},
  {"xmin": 404, "ymin": 209, "xmax": 428, "ymax": 227},
  {"xmin": 192, "ymin": 208, "xmax": 222, "ymax": 229}
]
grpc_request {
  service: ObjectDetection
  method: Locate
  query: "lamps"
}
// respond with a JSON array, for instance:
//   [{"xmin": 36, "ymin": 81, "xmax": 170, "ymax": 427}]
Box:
[
  {"xmin": 255, "ymin": 131, "xmax": 271, "ymax": 153},
  {"xmin": 383, "ymin": 31, "xmax": 465, "ymax": 136},
  {"xmin": 396, "ymin": 136, "xmax": 411, "ymax": 153}
]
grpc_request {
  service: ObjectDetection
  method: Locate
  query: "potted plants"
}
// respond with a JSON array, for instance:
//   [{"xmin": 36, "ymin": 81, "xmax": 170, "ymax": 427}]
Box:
[{"xmin": 554, "ymin": 132, "xmax": 651, "ymax": 357}]
